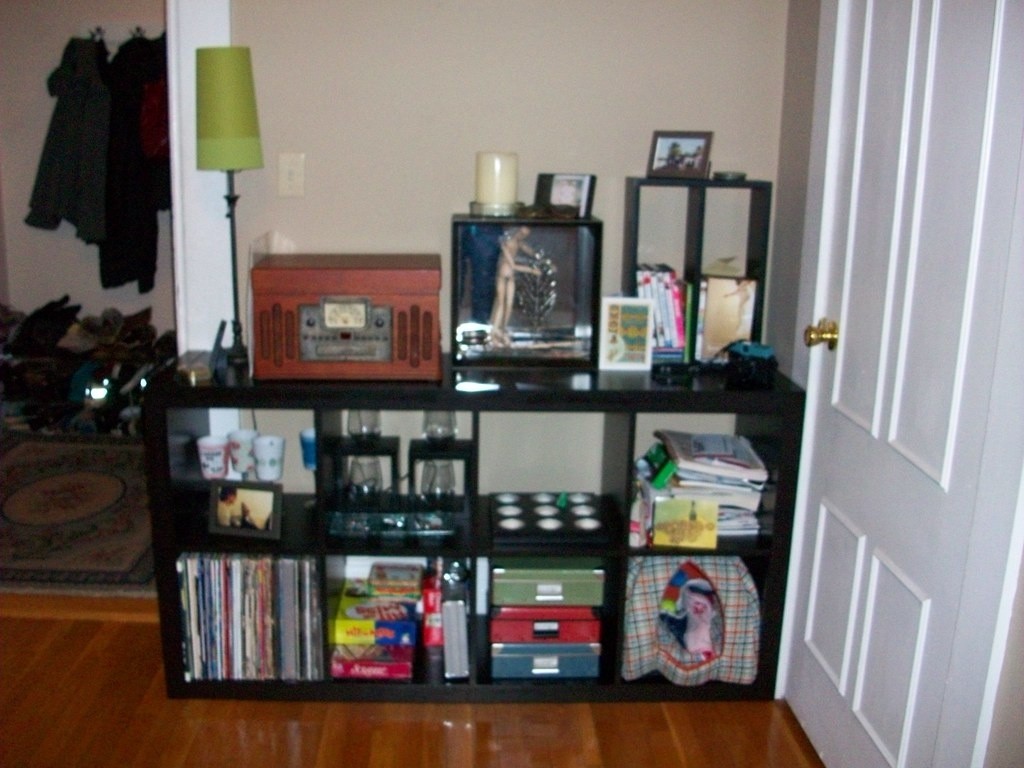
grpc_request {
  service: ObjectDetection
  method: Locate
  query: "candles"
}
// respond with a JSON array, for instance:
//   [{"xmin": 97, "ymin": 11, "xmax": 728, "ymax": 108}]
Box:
[{"xmin": 474, "ymin": 151, "xmax": 518, "ymax": 204}]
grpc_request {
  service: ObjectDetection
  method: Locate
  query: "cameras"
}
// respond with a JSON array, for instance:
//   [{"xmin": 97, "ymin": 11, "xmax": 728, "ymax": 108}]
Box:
[{"xmin": 728, "ymin": 341, "xmax": 780, "ymax": 394}]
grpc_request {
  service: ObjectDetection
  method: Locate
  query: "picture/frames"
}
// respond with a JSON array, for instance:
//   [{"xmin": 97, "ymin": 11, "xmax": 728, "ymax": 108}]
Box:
[
  {"xmin": 647, "ymin": 130, "xmax": 713, "ymax": 179},
  {"xmin": 206, "ymin": 478, "xmax": 281, "ymax": 539},
  {"xmin": 536, "ymin": 173, "xmax": 597, "ymax": 219}
]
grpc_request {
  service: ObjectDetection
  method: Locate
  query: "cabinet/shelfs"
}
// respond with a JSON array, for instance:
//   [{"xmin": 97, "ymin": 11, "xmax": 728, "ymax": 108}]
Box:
[
  {"xmin": 621, "ymin": 178, "xmax": 772, "ymax": 368},
  {"xmin": 140, "ymin": 347, "xmax": 807, "ymax": 703}
]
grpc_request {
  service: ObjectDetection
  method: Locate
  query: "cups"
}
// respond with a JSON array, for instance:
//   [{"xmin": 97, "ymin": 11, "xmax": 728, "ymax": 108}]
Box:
[
  {"xmin": 422, "ymin": 410, "xmax": 457, "ymax": 443},
  {"xmin": 420, "ymin": 459, "xmax": 456, "ymax": 506},
  {"xmin": 252, "ymin": 436, "xmax": 285, "ymax": 481},
  {"xmin": 347, "ymin": 410, "xmax": 382, "ymax": 438},
  {"xmin": 227, "ymin": 429, "xmax": 259, "ymax": 471},
  {"xmin": 197, "ymin": 436, "xmax": 229, "ymax": 479},
  {"xmin": 348, "ymin": 457, "xmax": 383, "ymax": 505},
  {"xmin": 300, "ymin": 428, "xmax": 316, "ymax": 470}
]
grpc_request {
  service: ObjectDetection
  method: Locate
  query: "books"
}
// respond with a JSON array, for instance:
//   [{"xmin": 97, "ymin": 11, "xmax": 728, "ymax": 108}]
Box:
[
  {"xmin": 182, "ymin": 552, "xmax": 326, "ymax": 683},
  {"xmin": 599, "ymin": 263, "xmax": 755, "ymax": 372},
  {"xmin": 654, "ymin": 428, "xmax": 766, "ymax": 535}
]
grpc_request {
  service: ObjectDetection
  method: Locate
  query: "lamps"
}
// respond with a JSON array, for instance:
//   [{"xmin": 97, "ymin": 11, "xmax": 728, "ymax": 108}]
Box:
[{"xmin": 196, "ymin": 47, "xmax": 263, "ymax": 363}]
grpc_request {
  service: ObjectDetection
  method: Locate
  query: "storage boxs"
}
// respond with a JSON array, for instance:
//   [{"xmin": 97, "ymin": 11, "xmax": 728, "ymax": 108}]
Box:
[
  {"xmin": 330, "ymin": 563, "xmax": 421, "ymax": 680},
  {"xmin": 490, "ymin": 606, "xmax": 603, "ymax": 643},
  {"xmin": 491, "ymin": 556, "xmax": 607, "ymax": 606},
  {"xmin": 490, "ymin": 643, "xmax": 603, "ymax": 680}
]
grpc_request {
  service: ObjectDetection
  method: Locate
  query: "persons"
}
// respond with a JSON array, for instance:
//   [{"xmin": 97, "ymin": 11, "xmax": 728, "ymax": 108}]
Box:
[
  {"xmin": 489, "ymin": 225, "xmax": 558, "ymax": 337},
  {"xmin": 217, "ymin": 487, "xmax": 238, "ymax": 524}
]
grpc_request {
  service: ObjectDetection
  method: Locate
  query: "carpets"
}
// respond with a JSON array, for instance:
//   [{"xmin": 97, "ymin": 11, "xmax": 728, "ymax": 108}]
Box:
[{"xmin": 0, "ymin": 420, "xmax": 157, "ymax": 599}]
